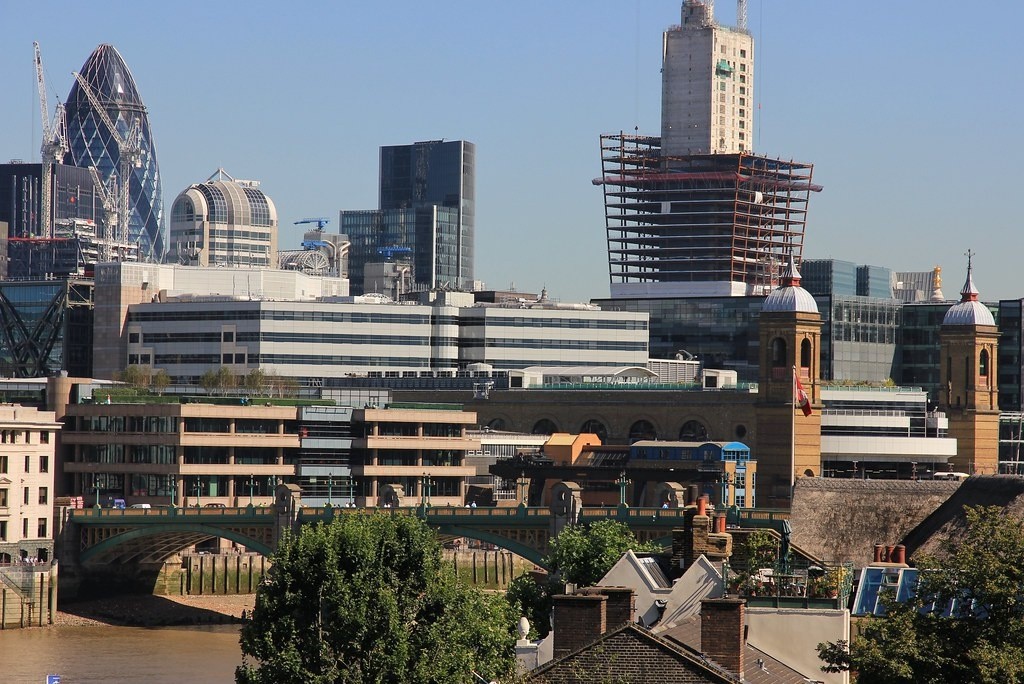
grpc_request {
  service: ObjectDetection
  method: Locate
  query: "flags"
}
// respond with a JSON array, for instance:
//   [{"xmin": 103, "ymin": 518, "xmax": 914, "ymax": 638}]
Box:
[{"xmin": 795, "ymin": 371, "xmax": 812, "ymax": 418}]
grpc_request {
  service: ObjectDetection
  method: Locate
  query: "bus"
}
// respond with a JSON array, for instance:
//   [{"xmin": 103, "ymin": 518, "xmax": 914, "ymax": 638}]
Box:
[{"xmin": 98, "ymin": 499, "xmax": 126, "ymax": 509}]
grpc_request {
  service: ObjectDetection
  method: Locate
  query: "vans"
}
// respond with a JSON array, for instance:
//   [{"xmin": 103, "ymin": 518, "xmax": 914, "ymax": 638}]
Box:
[{"xmin": 204, "ymin": 503, "xmax": 226, "ymax": 508}]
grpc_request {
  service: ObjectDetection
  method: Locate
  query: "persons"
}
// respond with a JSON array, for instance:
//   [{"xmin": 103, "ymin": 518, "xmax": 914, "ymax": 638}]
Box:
[
  {"xmin": 189, "ymin": 503, "xmax": 194, "ymax": 508},
  {"xmin": 323, "ymin": 501, "xmax": 357, "ymax": 509},
  {"xmin": 464, "ymin": 500, "xmax": 478, "ymax": 510},
  {"xmin": 382, "ymin": 502, "xmax": 392, "ymax": 509},
  {"xmin": 259, "ymin": 502, "xmax": 267, "ymax": 507},
  {"xmin": 112, "ymin": 501, "xmax": 124, "ymax": 509},
  {"xmin": 661, "ymin": 501, "xmax": 669, "ymax": 510},
  {"xmin": 241, "ymin": 395, "xmax": 250, "ymax": 406},
  {"xmin": 600, "ymin": 501, "xmax": 606, "ymax": 508},
  {"xmin": 12, "ymin": 555, "xmax": 44, "ymax": 566}
]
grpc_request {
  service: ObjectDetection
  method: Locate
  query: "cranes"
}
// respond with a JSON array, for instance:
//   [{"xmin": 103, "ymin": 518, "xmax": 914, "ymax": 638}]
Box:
[
  {"xmin": 31, "ymin": 40, "xmax": 69, "ymax": 238},
  {"xmin": 88, "ymin": 166, "xmax": 117, "ymax": 263},
  {"xmin": 72, "ymin": 71, "xmax": 148, "ymax": 261},
  {"xmin": 294, "ymin": 218, "xmax": 333, "ymax": 230}
]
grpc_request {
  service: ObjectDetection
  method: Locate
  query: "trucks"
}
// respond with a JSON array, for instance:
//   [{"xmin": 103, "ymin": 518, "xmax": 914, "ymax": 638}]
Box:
[{"xmin": 130, "ymin": 504, "xmax": 151, "ymax": 509}]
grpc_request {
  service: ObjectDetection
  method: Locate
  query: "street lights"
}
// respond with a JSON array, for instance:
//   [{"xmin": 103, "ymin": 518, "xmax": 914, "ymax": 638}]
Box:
[
  {"xmin": 96, "ymin": 476, "xmax": 100, "ymax": 505},
  {"xmin": 419, "ymin": 472, "xmax": 436, "ymax": 508},
  {"xmin": 193, "ymin": 475, "xmax": 205, "ymax": 507},
  {"xmin": 168, "ymin": 474, "xmax": 179, "ymax": 507},
  {"xmin": 615, "ymin": 470, "xmax": 631, "ymax": 509},
  {"xmin": 716, "ymin": 472, "xmax": 731, "ymax": 509},
  {"xmin": 246, "ymin": 474, "xmax": 258, "ymax": 502},
  {"xmin": 324, "ymin": 472, "xmax": 336, "ymax": 507},
  {"xmin": 517, "ymin": 470, "xmax": 527, "ymax": 508},
  {"xmin": 268, "ymin": 474, "xmax": 280, "ymax": 505},
  {"xmin": 346, "ymin": 474, "xmax": 358, "ymax": 508}
]
